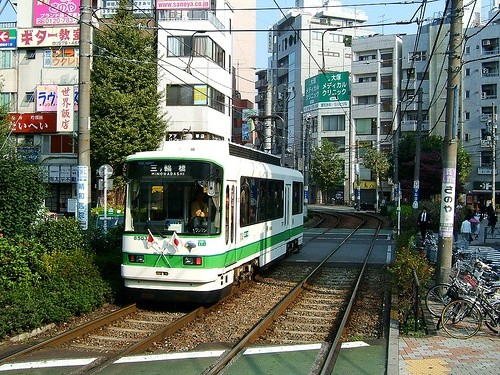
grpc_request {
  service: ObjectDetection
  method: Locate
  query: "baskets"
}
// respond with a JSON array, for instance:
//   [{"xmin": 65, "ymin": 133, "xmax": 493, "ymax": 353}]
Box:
[
  {"xmin": 441, "ymin": 266, "xmax": 451, "ymax": 278},
  {"xmin": 446, "ymin": 285, "xmax": 464, "ymax": 300}
]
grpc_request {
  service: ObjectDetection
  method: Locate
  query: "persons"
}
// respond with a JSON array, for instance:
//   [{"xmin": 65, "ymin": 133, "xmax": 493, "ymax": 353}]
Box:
[
  {"xmin": 489, "ymin": 213, "xmax": 498, "ymax": 235},
  {"xmin": 460, "ymin": 217, "xmax": 471, "ymax": 250},
  {"xmin": 189, "ymin": 193, "xmax": 209, "ymax": 223},
  {"xmin": 464, "ymin": 201, "xmax": 498, "ymax": 240},
  {"xmin": 332, "ymin": 196, "xmax": 386, "ymax": 212},
  {"xmin": 418, "ymin": 208, "xmax": 431, "ymax": 242},
  {"xmin": 453, "ymin": 202, "xmax": 463, "ymax": 241}
]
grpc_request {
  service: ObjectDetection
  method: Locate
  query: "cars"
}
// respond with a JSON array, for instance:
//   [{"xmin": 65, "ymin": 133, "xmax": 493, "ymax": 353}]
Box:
[{"xmin": 354, "ymin": 201, "xmax": 374, "ymax": 211}]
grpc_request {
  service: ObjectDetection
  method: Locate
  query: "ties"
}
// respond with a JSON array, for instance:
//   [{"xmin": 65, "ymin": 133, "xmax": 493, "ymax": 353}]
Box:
[{"xmin": 423, "ymin": 212, "xmax": 425, "ymax": 222}]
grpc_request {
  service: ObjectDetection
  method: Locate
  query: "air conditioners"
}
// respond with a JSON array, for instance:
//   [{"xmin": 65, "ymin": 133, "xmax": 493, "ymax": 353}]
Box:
[{"xmin": 483, "ymin": 40, "xmax": 490, "ymax": 45}]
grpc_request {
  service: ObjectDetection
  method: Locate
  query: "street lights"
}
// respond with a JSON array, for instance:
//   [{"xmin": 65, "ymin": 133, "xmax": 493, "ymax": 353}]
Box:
[{"xmin": 247, "ymin": 114, "xmax": 286, "ymax": 167}]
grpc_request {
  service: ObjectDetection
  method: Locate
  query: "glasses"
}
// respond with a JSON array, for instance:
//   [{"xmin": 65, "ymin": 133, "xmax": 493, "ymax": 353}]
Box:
[{"xmin": 423, "ymin": 208, "xmax": 426, "ymax": 210}]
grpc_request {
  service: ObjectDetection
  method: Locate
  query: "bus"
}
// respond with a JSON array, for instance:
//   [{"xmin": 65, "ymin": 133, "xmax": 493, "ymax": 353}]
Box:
[{"xmin": 121, "ymin": 140, "xmax": 304, "ymax": 308}]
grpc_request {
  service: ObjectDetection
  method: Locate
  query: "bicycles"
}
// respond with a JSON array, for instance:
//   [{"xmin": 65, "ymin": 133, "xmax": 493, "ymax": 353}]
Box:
[
  {"xmin": 485, "ymin": 226, "xmax": 497, "ymax": 239},
  {"xmin": 424, "ymin": 229, "xmax": 500, "ymax": 339}
]
